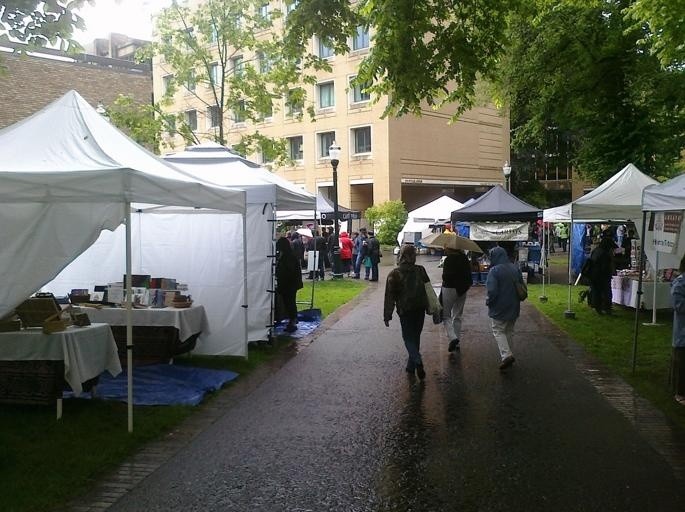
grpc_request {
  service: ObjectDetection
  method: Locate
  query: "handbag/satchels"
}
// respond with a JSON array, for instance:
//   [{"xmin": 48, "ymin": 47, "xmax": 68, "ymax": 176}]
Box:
[
  {"xmin": 514, "ymin": 281, "xmax": 527, "ymax": 301},
  {"xmin": 364, "ymin": 255, "xmax": 372, "ymax": 268},
  {"xmin": 420, "ymin": 266, "xmax": 443, "ymax": 315},
  {"xmin": 432, "ymin": 294, "xmax": 442, "ymax": 324}
]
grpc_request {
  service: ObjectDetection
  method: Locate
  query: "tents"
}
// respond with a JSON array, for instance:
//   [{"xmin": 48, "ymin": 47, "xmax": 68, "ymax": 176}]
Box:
[
  {"xmin": 450, "ymin": 184, "xmax": 550, "ymax": 282},
  {"xmin": 276, "ymin": 191, "xmax": 360, "ymax": 234},
  {"xmin": 398, "ymin": 195, "xmax": 465, "ymax": 254}
]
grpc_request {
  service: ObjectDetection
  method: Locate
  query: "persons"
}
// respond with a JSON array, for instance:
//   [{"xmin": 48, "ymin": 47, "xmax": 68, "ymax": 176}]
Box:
[
  {"xmin": 384, "ymin": 244, "xmax": 432, "ymax": 379},
  {"xmin": 432, "ymin": 247, "xmax": 474, "ymax": 353},
  {"xmin": 538, "ymin": 220, "xmax": 634, "ymax": 253},
  {"xmin": 484, "ymin": 245, "xmax": 523, "ymax": 370},
  {"xmin": 286, "ymin": 226, "xmax": 380, "ymax": 280},
  {"xmin": 275, "ymin": 237, "xmax": 302, "ymax": 332},
  {"xmin": 588, "ymin": 238, "xmax": 619, "ymax": 317},
  {"xmin": 667, "ymin": 254, "xmax": 684, "ymax": 403}
]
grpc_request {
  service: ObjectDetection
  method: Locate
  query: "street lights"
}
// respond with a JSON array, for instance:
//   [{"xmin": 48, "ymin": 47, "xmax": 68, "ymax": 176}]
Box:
[
  {"xmin": 329, "ymin": 139, "xmax": 345, "ymax": 280},
  {"xmin": 503, "ymin": 160, "xmax": 512, "ymax": 191}
]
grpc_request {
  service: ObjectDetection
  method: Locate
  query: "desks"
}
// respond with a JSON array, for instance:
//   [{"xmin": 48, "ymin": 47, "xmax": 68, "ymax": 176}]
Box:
[
  {"xmin": 609, "ymin": 271, "xmax": 682, "ymax": 313},
  {"xmin": 56, "ymin": 302, "xmax": 205, "ymax": 369},
  {"xmin": 1, "ymin": 321, "xmax": 126, "ymax": 422}
]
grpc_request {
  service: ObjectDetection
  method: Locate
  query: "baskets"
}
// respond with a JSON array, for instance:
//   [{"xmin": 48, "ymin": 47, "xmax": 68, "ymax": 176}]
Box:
[{"xmin": 169, "ymin": 298, "xmax": 194, "ymax": 308}]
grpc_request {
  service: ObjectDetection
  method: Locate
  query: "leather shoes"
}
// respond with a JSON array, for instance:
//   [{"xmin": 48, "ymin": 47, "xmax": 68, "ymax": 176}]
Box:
[
  {"xmin": 448, "ymin": 339, "xmax": 459, "ymax": 352},
  {"xmin": 498, "ymin": 355, "xmax": 515, "ymax": 369}
]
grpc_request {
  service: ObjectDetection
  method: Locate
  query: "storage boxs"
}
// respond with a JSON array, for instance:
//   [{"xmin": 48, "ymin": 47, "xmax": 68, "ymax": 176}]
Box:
[{"xmin": 471, "ymin": 242, "xmax": 542, "ymax": 284}]
